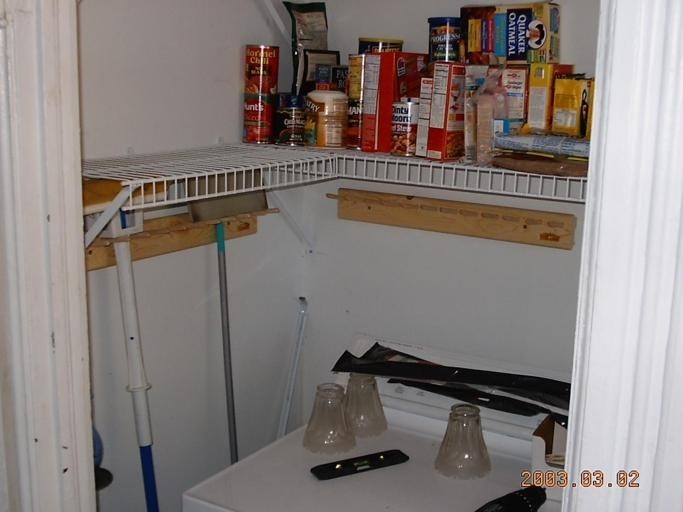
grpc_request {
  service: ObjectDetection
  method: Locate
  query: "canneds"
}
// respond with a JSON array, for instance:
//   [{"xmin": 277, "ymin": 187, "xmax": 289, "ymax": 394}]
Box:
[
  {"xmin": 359, "ymin": 37, "xmax": 403, "ymax": 54},
  {"xmin": 243, "ymin": 44, "xmax": 349, "ymax": 149},
  {"xmin": 391, "ymin": 102, "xmax": 420, "ymax": 156},
  {"xmin": 428, "ymin": 17, "xmax": 465, "ymax": 62}
]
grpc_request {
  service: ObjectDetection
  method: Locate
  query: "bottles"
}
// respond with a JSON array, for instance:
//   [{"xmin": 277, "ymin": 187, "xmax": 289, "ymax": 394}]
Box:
[
  {"xmin": 436, "ymin": 405, "xmax": 491, "ymax": 481},
  {"xmin": 302, "ymin": 383, "xmax": 357, "ymax": 454},
  {"xmin": 344, "ymin": 370, "xmax": 387, "ymax": 437}
]
